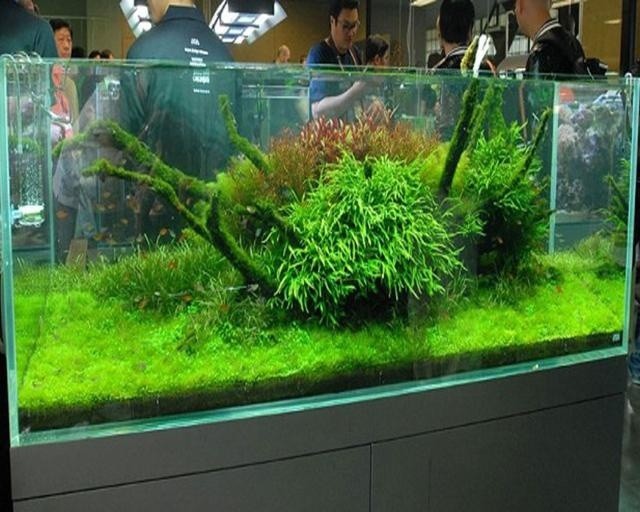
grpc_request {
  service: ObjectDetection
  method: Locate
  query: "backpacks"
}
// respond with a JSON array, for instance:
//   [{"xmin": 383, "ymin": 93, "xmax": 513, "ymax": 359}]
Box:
[{"xmin": 537, "ymin": 29, "xmax": 609, "ymax": 75}]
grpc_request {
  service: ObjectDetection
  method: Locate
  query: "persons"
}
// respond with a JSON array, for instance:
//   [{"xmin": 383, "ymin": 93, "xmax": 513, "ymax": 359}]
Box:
[{"xmin": 1, "ymin": 0, "xmax": 590, "ymax": 262}]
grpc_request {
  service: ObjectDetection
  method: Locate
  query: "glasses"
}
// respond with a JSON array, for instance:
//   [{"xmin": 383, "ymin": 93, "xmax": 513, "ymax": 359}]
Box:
[{"xmin": 337, "ymin": 19, "xmax": 360, "ymax": 30}]
